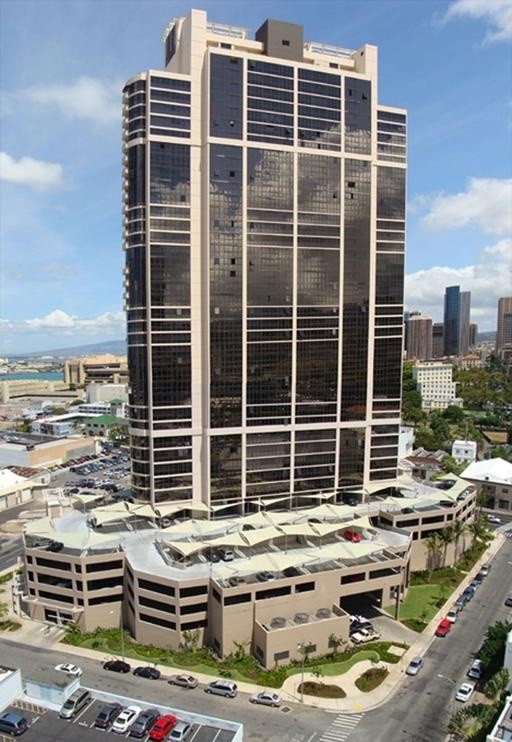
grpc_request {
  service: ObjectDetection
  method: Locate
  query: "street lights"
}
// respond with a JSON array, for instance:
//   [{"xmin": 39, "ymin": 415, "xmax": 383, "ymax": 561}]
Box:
[
  {"xmin": 110, "ymin": 609, "xmax": 125, "ymax": 663},
  {"xmin": 297, "ymin": 643, "xmax": 305, "ymax": 703},
  {"xmin": 435, "ymin": 673, "xmax": 457, "ymax": 742}
]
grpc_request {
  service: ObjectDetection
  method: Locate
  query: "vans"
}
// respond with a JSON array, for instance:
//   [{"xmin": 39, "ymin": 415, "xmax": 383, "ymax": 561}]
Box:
[{"xmin": 60, "ymin": 688, "xmax": 92, "ymax": 719}]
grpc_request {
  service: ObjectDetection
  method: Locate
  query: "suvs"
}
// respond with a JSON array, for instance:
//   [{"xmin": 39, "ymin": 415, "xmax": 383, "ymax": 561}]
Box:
[
  {"xmin": 0, "ymin": 712, "xmax": 27, "ymax": 737},
  {"xmin": 204, "ymin": 681, "xmax": 238, "ymax": 698}
]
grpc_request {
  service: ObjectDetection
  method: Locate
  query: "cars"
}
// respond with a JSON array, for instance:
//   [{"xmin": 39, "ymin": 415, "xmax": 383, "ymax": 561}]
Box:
[
  {"xmin": 133, "ymin": 668, "xmax": 160, "ymax": 680},
  {"xmin": 96, "ymin": 704, "xmax": 122, "ymax": 728},
  {"xmin": 168, "ymin": 721, "xmax": 194, "ymax": 742},
  {"xmin": 60, "ymin": 441, "xmax": 132, "ymax": 500},
  {"xmin": 112, "ymin": 706, "xmax": 139, "ymax": 734},
  {"xmin": 53, "ymin": 663, "xmax": 83, "ymax": 677},
  {"xmin": 103, "ymin": 660, "xmax": 130, "ymax": 674},
  {"xmin": 128, "ymin": 709, "xmax": 160, "ymax": 739},
  {"xmin": 344, "ymin": 507, "xmax": 512, "ymax": 703},
  {"xmin": 205, "ymin": 548, "xmax": 277, "ymax": 586},
  {"xmin": 149, "ymin": 714, "xmax": 177, "ymax": 742},
  {"xmin": 168, "ymin": 676, "xmax": 199, "ymax": 689},
  {"xmin": 250, "ymin": 692, "xmax": 282, "ymax": 707}
]
